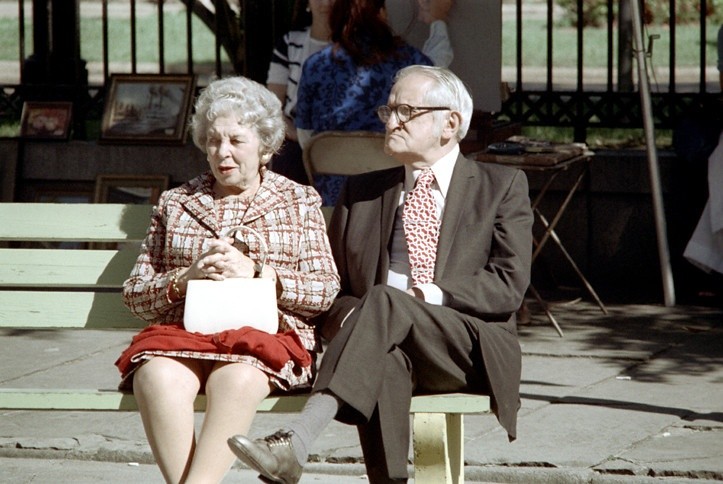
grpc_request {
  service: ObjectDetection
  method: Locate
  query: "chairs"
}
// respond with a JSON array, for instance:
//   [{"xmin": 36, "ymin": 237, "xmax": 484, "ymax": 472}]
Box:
[{"xmin": 303, "ymin": 130, "xmax": 405, "ymax": 231}]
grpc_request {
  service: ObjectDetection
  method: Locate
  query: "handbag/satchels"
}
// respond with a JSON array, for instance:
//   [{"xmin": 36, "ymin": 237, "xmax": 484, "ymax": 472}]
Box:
[{"xmin": 182, "ymin": 227, "xmax": 280, "ymax": 335}]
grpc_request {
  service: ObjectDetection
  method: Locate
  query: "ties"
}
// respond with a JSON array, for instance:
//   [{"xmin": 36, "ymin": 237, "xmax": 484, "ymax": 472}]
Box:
[{"xmin": 402, "ymin": 168, "xmax": 441, "ymax": 285}]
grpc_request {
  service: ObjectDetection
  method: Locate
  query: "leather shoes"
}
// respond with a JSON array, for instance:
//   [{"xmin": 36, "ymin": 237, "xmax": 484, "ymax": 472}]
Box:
[{"xmin": 226, "ymin": 430, "xmax": 303, "ymax": 484}]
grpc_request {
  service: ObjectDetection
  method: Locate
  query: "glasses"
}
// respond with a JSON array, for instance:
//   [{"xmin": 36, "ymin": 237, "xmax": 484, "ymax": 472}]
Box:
[{"xmin": 376, "ymin": 104, "xmax": 451, "ymax": 124}]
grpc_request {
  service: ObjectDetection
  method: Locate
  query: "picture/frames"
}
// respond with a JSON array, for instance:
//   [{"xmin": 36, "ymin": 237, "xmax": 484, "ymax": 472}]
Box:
[
  {"xmin": 88, "ymin": 173, "xmax": 169, "ymax": 250},
  {"xmin": 97, "ymin": 73, "xmax": 198, "ymax": 145},
  {"xmin": 22, "ymin": 191, "xmax": 91, "ymax": 249},
  {"xmin": 20, "ymin": 101, "xmax": 73, "ymax": 143}
]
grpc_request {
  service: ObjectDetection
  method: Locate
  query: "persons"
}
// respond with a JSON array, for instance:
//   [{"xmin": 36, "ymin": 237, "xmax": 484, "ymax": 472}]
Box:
[
  {"xmin": 226, "ymin": 66, "xmax": 534, "ymax": 484},
  {"xmin": 296, "ymin": 0, "xmax": 454, "ymax": 206},
  {"xmin": 115, "ymin": 75, "xmax": 341, "ymax": 484},
  {"xmin": 265, "ymin": 0, "xmax": 338, "ymax": 186}
]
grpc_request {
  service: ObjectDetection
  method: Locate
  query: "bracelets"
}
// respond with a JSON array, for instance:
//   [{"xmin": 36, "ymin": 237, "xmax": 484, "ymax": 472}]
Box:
[{"xmin": 172, "ymin": 270, "xmax": 185, "ymax": 300}]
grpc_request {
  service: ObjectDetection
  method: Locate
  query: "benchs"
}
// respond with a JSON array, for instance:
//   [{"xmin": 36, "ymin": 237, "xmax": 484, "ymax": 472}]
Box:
[{"xmin": 0, "ymin": 203, "xmax": 490, "ymax": 484}]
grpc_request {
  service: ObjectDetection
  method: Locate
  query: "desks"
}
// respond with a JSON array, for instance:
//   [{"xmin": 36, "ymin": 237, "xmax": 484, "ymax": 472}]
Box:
[{"xmin": 465, "ymin": 151, "xmax": 607, "ymax": 338}]
